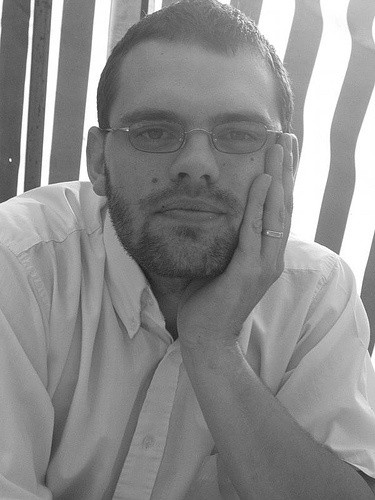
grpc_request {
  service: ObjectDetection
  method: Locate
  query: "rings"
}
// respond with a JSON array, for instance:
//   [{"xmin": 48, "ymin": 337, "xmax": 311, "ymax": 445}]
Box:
[{"xmin": 261, "ymin": 227, "xmax": 284, "ymax": 239}]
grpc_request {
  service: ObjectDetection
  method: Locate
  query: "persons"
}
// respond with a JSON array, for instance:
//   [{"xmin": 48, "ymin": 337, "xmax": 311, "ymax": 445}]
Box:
[{"xmin": 0, "ymin": 0, "xmax": 375, "ymax": 500}]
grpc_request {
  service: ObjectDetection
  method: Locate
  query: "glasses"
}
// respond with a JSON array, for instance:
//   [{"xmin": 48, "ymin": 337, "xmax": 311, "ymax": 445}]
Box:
[{"xmin": 103, "ymin": 117, "xmax": 283, "ymax": 154}]
